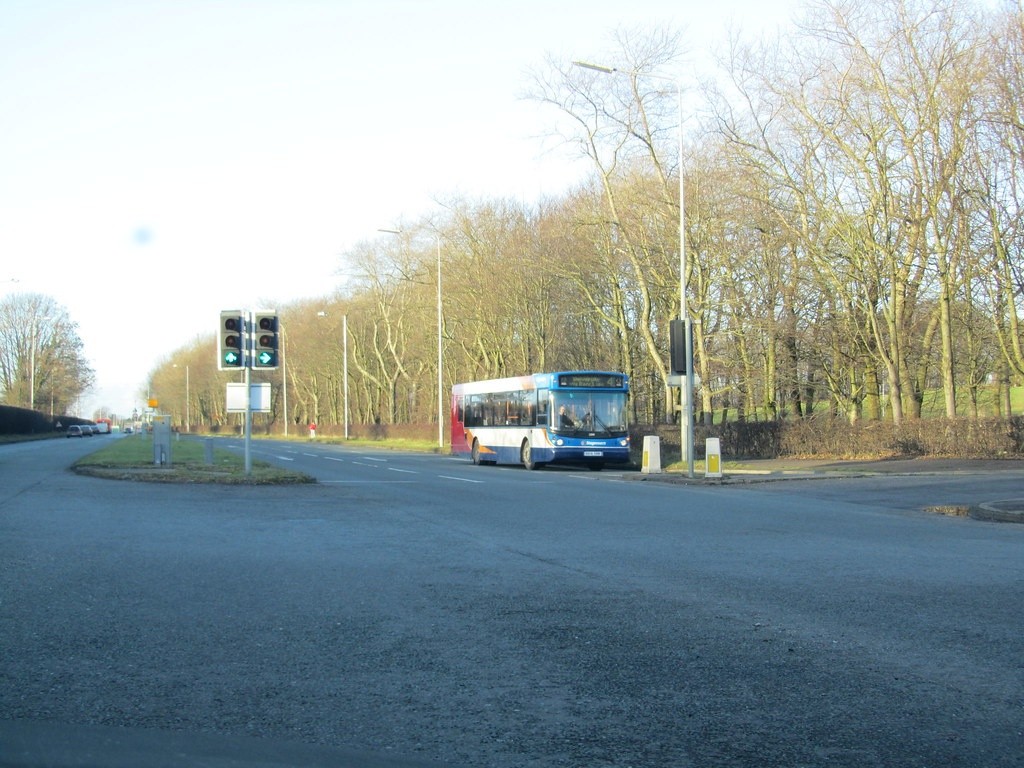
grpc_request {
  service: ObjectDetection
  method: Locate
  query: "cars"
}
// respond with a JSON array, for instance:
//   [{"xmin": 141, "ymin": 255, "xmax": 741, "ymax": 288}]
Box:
[
  {"xmin": 80, "ymin": 425, "xmax": 92, "ymax": 436},
  {"xmin": 67, "ymin": 425, "xmax": 83, "ymax": 438},
  {"xmin": 123, "ymin": 427, "xmax": 133, "ymax": 434},
  {"xmin": 90, "ymin": 426, "xmax": 100, "ymax": 433}
]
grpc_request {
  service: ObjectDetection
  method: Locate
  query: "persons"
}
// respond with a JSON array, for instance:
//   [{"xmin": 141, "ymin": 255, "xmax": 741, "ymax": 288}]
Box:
[
  {"xmin": 474, "ymin": 403, "xmax": 499, "ymax": 423},
  {"xmin": 465, "ymin": 406, "xmax": 471, "ymax": 426},
  {"xmin": 560, "ymin": 405, "xmax": 574, "ymax": 432},
  {"xmin": 309, "ymin": 421, "xmax": 316, "ymax": 437},
  {"xmin": 56, "ymin": 420, "xmax": 62, "ymax": 431}
]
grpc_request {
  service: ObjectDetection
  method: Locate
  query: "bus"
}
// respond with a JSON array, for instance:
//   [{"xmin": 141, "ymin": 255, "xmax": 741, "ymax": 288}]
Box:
[
  {"xmin": 450, "ymin": 371, "xmax": 631, "ymax": 470},
  {"xmin": 98, "ymin": 418, "xmax": 110, "ymax": 433}
]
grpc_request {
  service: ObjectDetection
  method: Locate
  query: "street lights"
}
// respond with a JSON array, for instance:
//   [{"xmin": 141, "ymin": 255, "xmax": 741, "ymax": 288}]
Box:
[
  {"xmin": 572, "ymin": 59, "xmax": 694, "ymax": 475},
  {"xmin": 30, "ymin": 316, "xmax": 51, "ymax": 410},
  {"xmin": 379, "ymin": 229, "xmax": 443, "ymax": 447},
  {"xmin": 317, "ymin": 312, "xmax": 349, "ymax": 440},
  {"xmin": 173, "ymin": 364, "xmax": 190, "ymax": 432}
]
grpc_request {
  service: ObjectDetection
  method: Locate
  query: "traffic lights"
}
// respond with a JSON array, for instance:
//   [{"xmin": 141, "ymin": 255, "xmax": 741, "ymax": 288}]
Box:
[
  {"xmin": 251, "ymin": 310, "xmax": 279, "ymax": 370},
  {"xmin": 218, "ymin": 308, "xmax": 246, "ymax": 371}
]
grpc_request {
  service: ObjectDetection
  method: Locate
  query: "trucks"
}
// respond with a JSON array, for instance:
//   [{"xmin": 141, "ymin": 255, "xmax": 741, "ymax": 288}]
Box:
[{"xmin": 96, "ymin": 423, "xmax": 108, "ymax": 433}]
